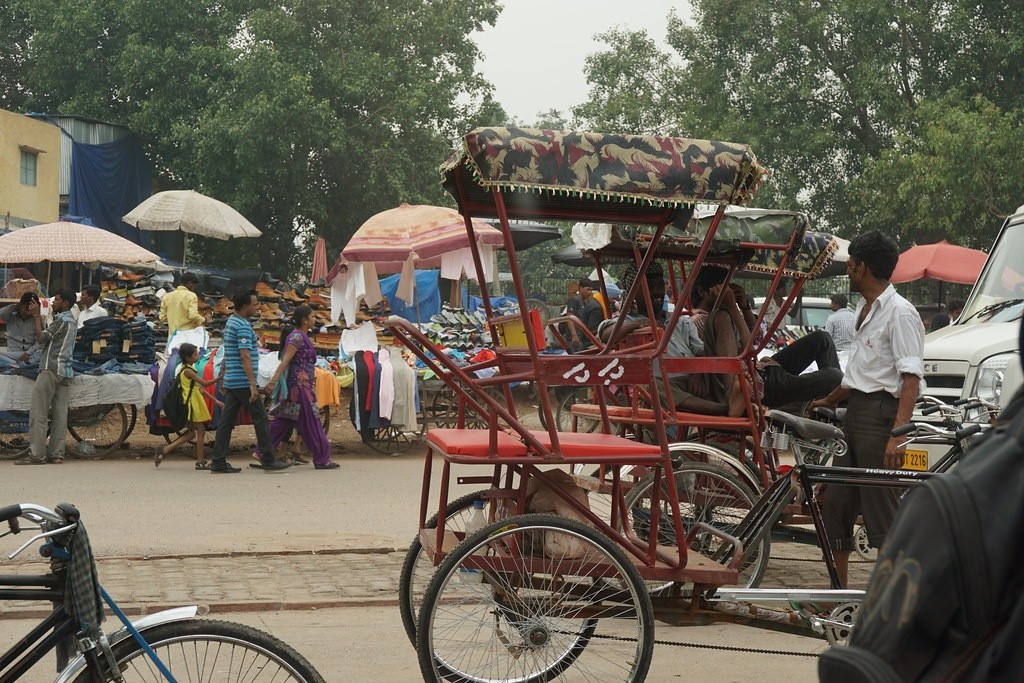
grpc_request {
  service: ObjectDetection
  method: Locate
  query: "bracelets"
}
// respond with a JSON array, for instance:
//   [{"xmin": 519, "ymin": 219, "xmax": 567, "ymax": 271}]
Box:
[{"xmin": 269, "ymin": 381, "xmax": 276, "ymax": 384}]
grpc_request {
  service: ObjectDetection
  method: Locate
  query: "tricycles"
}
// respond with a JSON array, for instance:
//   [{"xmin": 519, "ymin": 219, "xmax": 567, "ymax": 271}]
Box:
[{"xmin": 382, "ymin": 125, "xmax": 1001, "ymax": 683}]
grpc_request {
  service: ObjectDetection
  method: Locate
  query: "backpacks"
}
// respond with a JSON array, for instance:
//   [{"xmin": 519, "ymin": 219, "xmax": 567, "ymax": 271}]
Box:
[
  {"xmin": 818, "ymin": 385, "xmax": 1024, "ymax": 683},
  {"xmin": 162, "ymin": 367, "xmax": 197, "ymax": 430}
]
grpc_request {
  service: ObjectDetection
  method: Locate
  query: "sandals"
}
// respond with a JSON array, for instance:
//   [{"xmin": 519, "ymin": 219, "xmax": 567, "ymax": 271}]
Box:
[
  {"xmin": 154, "ymin": 445, "xmax": 167, "ymax": 467},
  {"xmin": 195, "ymin": 460, "xmax": 210, "ymax": 470}
]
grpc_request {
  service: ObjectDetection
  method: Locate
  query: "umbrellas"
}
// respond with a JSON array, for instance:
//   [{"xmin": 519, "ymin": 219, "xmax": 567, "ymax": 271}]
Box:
[
  {"xmin": 0, "ymin": 221, "xmax": 162, "ymax": 296},
  {"xmin": 312, "ymin": 236, "xmax": 328, "ymax": 283},
  {"xmin": 889, "ymin": 237, "xmax": 1024, "ymax": 316},
  {"xmin": 122, "ymin": 189, "xmax": 264, "ymax": 269},
  {"xmin": 326, "ymin": 202, "xmax": 506, "ymax": 331},
  {"xmin": 733, "ymin": 231, "xmax": 853, "ymax": 329}
]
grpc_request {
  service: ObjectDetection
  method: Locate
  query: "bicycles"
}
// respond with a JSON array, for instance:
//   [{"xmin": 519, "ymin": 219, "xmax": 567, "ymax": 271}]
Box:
[{"xmin": 0, "ymin": 502, "xmax": 328, "ymax": 683}]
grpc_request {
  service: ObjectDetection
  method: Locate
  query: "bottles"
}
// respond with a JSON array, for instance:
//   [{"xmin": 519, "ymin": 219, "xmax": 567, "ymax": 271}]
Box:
[{"xmin": 460, "ymin": 498, "xmax": 489, "ymax": 584}]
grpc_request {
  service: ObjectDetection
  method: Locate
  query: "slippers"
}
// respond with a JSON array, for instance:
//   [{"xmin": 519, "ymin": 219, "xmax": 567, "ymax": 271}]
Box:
[
  {"xmin": 250, "ymin": 464, "xmax": 263, "ymax": 468},
  {"xmin": 316, "ymin": 462, "xmax": 340, "ymax": 469},
  {"xmin": 789, "ymin": 600, "xmax": 824, "ymax": 615}
]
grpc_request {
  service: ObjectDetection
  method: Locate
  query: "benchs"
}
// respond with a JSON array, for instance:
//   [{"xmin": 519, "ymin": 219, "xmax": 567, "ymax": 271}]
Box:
[{"xmin": 386, "ymin": 312, "xmax": 757, "ymax": 563}]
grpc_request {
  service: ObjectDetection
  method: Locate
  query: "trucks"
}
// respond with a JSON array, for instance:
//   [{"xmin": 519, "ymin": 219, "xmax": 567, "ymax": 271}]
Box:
[{"xmin": 902, "ymin": 203, "xmax": 1024, "ymax": 476}]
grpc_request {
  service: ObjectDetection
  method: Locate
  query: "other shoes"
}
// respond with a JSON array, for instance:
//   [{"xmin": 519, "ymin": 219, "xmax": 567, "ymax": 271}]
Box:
[
  {"xmin": 277, "ymin": 456, "xmax": 299, "ymax": 466},
  {"xmin": 15, "ymin": 454, "xmax": 46, "ymax": 464},
  {"xmin": 10, "ymin": 437, "xmax": 30, "ymax": 445},
  {"xmin": 262, "ymin": 463, "xmax": 291, "ymax": 472},
  {"xmin": 211, "ymin": 463, "xmax": 241, "ymax": 473},
  {"xmin": 46, "ymin": 457, "xmax": 62, "ymax": 464},
  {"xmin": 98, "ymin": 266, "xmax": 517, "ymax": 370},
  {"xmin": 287, "ymin": 452, "xmax": 308, "ymax": 465}
]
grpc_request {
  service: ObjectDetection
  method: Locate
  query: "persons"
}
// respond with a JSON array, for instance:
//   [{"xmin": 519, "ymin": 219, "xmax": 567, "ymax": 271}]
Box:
[
  {"xmin": 565, "ymin": 231, "xmax": 967, "ymax": 615},
  {"xmin": 817, "ymin": 309, "xmax": 1024, "ymax": 683},
  {"xmin": 0, "ymin": 285, "xmax": 108, "ymax": 465},
  {"xmin": 159, "ymin": 272, "xmax": 207, "ymax": 338},
  {"xmin": 155, "ymin": 291, "xmax": 340, "ymax": 474}
]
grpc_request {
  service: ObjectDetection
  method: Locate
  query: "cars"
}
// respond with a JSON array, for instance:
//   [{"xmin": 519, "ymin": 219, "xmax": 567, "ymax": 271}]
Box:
[{"xmin": 735, "ymin": 296, "xmax": 858, "ymax": 327}]
